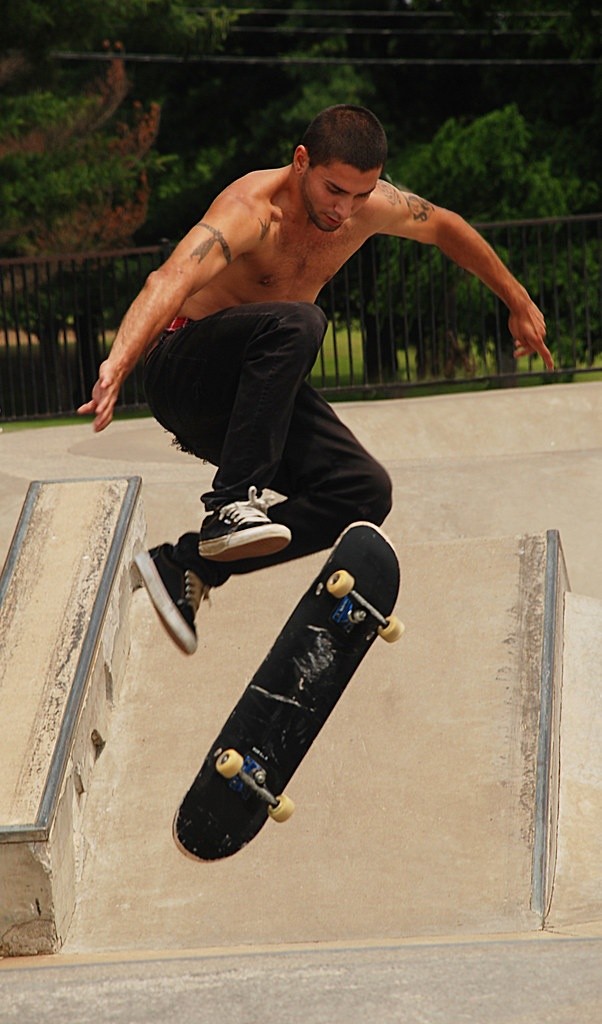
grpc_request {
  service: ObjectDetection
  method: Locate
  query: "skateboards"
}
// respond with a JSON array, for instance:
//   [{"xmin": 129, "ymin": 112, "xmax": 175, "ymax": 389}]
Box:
[{"xmin": 172, "ymin": 520, "xmax": 404, "ymax": 865}]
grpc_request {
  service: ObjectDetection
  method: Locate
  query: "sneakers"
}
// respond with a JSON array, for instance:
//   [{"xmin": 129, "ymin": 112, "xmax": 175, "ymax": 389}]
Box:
[
  {"xmin": 196, "ymin": 484, "xmax": 294, "ymax": 563},
  {"xmin": 134, "ymin": 542, "xmax": 212, "ymax": 656}
]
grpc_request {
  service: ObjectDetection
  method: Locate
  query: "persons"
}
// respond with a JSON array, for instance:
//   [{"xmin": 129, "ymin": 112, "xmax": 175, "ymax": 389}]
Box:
[{"xmin": 75, "ymin": 101, "xmax": 557, "ymax": 654}]
